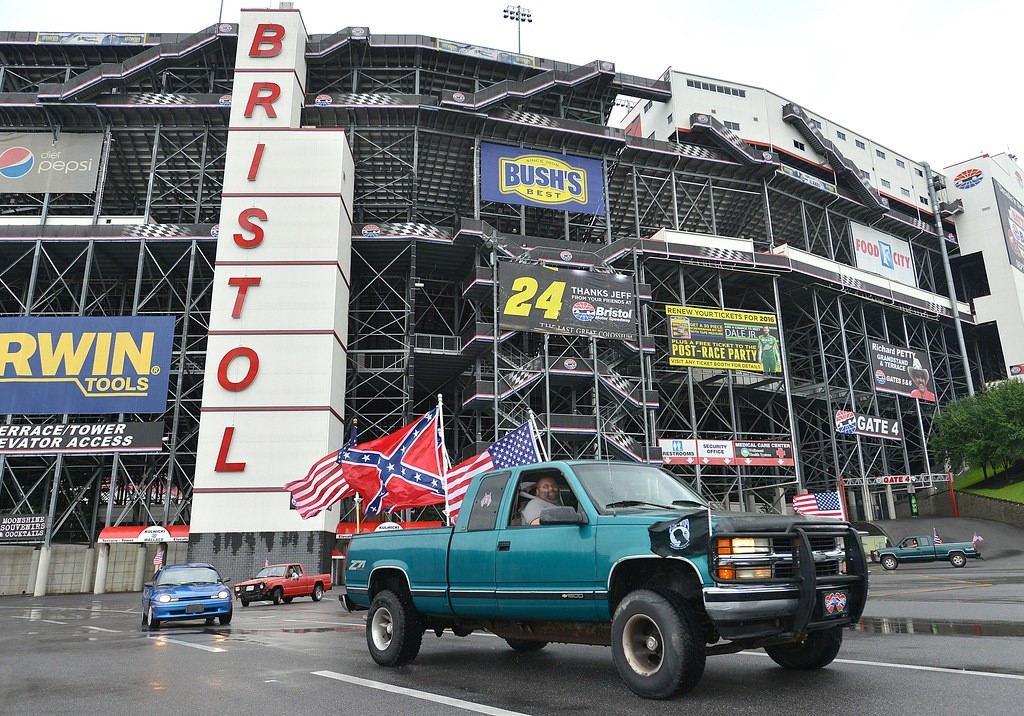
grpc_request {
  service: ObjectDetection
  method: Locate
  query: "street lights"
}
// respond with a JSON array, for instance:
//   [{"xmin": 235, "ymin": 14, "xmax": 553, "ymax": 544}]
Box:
[{"xmin": 502, "ymin": 4, "xmax": 532, "ymax": 55}]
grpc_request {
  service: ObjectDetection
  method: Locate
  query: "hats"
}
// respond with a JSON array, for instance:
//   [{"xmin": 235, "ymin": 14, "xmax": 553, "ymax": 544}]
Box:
[{"xmin": 907, "ymin": 358, "xmax": 930, "ymax": 386}]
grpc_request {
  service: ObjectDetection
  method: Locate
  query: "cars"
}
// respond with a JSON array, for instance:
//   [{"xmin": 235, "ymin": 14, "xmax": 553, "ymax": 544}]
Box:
[{"xmin": 141, "ymin": 561, "xmax": 233, "ymax": 630}]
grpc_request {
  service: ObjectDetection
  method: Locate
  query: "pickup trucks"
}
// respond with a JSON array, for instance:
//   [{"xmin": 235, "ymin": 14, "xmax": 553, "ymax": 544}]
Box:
[
  {"xmin": 339, "ymin": 459, "xmax": 870, "ymax": 700},
  {"xmin": 232, "ymin": 561, "xmax": 332, "ymax": 606},
  {"xmin": 869, "ymin": 534, "xmax": 984, "ymax": 571}
]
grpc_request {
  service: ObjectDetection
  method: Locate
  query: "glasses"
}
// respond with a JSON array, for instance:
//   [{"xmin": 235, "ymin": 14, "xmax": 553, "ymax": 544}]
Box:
[{"xmin": 916, "ymin": 374, "xmax": 924, "ymax": 379}]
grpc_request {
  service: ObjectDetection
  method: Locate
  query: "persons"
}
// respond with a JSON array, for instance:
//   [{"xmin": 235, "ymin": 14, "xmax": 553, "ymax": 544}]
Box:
[
  {"xmin": 758, "ymin": 324, "xmax": 780, "ymax": 371},
  {"xmin": 521, "ymin": 477, "xmax": 563, "ymax": 526},
  {"xmin": 909, "ymin": 540, "xmax": 918, "ymax": 547},
  {"xmin": 288, "ymin": 568, "xmax": 298, "ymax": 581},
  {"xmin": 907, "ymin": 358, "xmax": 935, "ymax": 401}
]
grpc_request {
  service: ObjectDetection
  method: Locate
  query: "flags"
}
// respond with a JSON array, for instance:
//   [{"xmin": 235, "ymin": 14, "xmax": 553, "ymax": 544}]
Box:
[
  {"xmin": 341, "ymin": 407, "xmax": 446, "ymax": 517},
  {"xmin": 973, "ymin": 535, "xmax": 984, "ymax": 542},
  {"xmin": 445, "ymin": 420, "xmax": 542, "ymax": 525},
  {"xmin": 793, "ymin": 491, "xmax": 843, "ymax": 518},
  {"xmin": 647, "ymin": 510, "xmax": 708, "ymax": 558},
  {"xmin": 283, "ymin": 439, "xmax": 356, "ymax": 520},
  {"xmin": 265, "ymin": 559, "xmax": 269, "ymax": 567},
  {"xmin": 934, "ymin": 527, "xmax": 942, "ymax": 544},
  {"xmin": 153, "ymin": 551, "xmax": 164, "ymax": 566}
]
